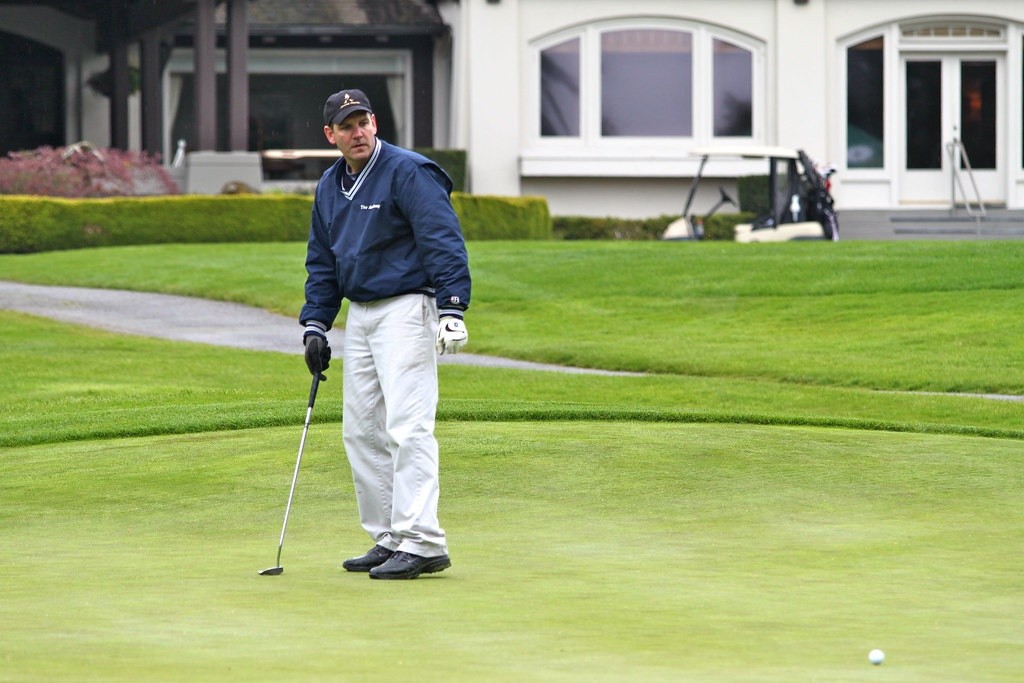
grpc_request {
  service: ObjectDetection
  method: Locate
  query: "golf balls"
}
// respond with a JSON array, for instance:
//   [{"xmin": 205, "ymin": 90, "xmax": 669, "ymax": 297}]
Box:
[{"xmin": 868, "ymin": 648, "xmax": 885, "ymax": 665}]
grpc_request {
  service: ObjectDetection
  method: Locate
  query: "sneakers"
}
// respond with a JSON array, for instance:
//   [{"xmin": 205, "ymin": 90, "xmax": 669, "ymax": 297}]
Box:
[
  {"xmin": 369, "ymin": 550, "xmax": 452, "ymax": 580},
  {"xmin": 342, "ymin": 545, "xmax": 394, "ymax": 572}
]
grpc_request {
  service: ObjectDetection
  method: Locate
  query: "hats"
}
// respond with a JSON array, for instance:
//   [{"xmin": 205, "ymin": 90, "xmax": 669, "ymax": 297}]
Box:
[{"xmin": 324, "ymin": 89, "xmax": 372, "ymax": 125}]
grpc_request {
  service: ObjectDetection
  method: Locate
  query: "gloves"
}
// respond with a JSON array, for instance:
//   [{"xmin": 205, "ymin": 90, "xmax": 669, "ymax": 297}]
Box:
[
  {"xmin": 303, "ymin": 336, "xmax": 332, "ymax": 381},
  {"xmin": 436, "ymin": 315, "xmax": 468, "ymax": 356}
]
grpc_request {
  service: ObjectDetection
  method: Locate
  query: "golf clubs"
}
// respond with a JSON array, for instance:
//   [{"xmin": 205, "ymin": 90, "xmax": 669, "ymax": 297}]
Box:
[{"xmin": 258, "ymin": 340, "xmax": 329, "ymax": 576}]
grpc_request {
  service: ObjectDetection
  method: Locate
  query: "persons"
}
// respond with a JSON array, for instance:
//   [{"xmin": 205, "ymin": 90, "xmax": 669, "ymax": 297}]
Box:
[{"xmin": 298, "ymin": 90, "xmax": 474, "ymax": 580}]
